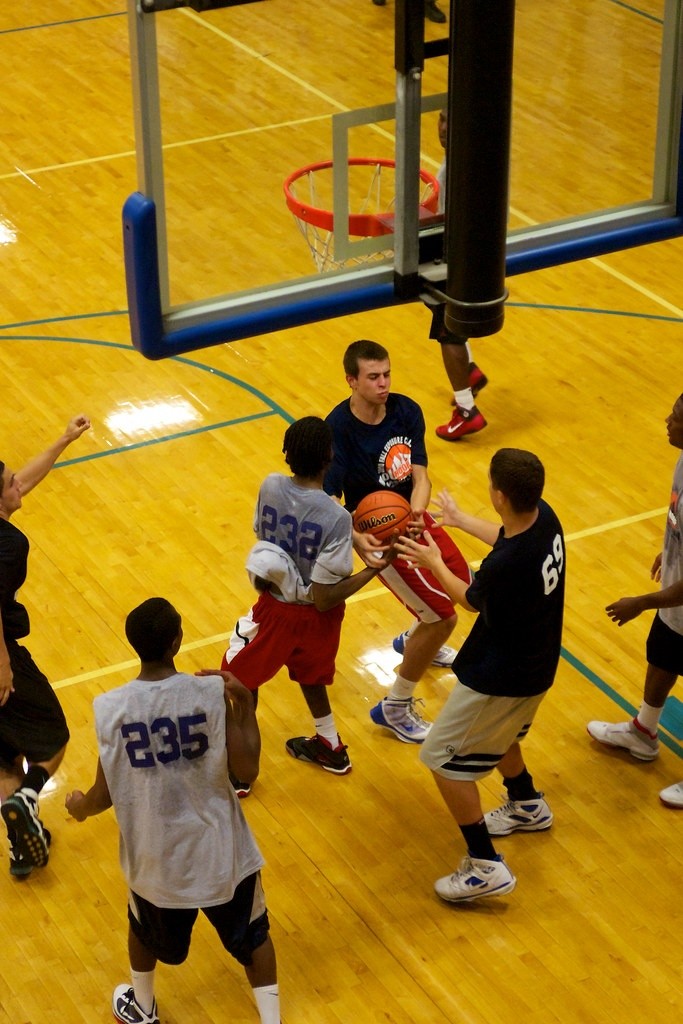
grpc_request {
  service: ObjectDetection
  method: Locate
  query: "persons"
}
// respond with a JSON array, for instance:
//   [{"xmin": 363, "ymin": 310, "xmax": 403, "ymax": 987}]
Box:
[
  {"xmin": 0, "ymin": 414, "xmax": 92, "ymax": 876},
  {"xmin": 316, "ymin": 340, "xmax": 475, "ymax": 746},
  {"xmin": 586, "ymin": 395, "xmax": 683, "ymax": 809},
  {"xmin": 391, "ymin": 447, "xmax": 566, "ymax": 904},
  {"xmin": 413, "ymin": 112, "xmax": 487, "ymax": 438},
  {"xmin": 371, "ymin": 0, "xmax": 446, "ymax": 23},
  {"xmin": 66, "ymin": 598, "xmax": 283, "ymax": 1023},
  {"xmin": 224, "ymin": 416, "xmax": 407, "ymax": 797}
]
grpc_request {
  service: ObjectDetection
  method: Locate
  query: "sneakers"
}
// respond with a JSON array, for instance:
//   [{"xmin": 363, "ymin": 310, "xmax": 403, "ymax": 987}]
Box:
[
  {"xmin": 436, "ymin": 405, "xmax": 488, "ymax": 441},
  {"xmin": 227, "ymin": 769, "xmax": 251, "ymax": 798},
  {"xmin": 369, "ymin": 694, "xmax": 434, "ymax": 745},
  {"xmin": 587, "ymin": 720, "xmax": 660, "ymax": 762},
  {"xmin": 285, "ymin": 732, "xmax": 353, "ymax": 777},
  {"xmin": 484, "ymin": 790, "xmax": 554, "ymax": 838},
  {"xmin": 659, "ymin": 781, "xmax": 683, "ymax": 809},
  {"xmin": 2, "ymin": 788, "xmax": 51, "ymax": 876},
  {"xmin": 450, "ymin": 361, "xmax": 487, "ymax": 406},
  {"xmin": 112, "ymin": 984, "xmax": 160, "ymax": 1024},
  {"xmin": 433, "ymin": 850, "xmax": 517, "ymax": 904},
  {"xmin": 393, "ymin": 630, "xmax": 459, "ymax": 668}
]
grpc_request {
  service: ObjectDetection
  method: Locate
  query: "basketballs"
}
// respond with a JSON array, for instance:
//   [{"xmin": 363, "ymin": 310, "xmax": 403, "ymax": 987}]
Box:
[{"xmin": 354, "ymin": 490, "xmax": 415, "ymax": 545}]
